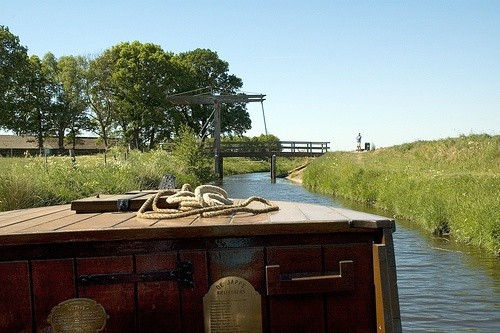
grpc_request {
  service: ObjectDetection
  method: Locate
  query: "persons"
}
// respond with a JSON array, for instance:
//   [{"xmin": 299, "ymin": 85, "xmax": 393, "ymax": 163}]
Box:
[{"xmin": 356, "ymin": 133, "xmax": 363, "ymax": 151}]
